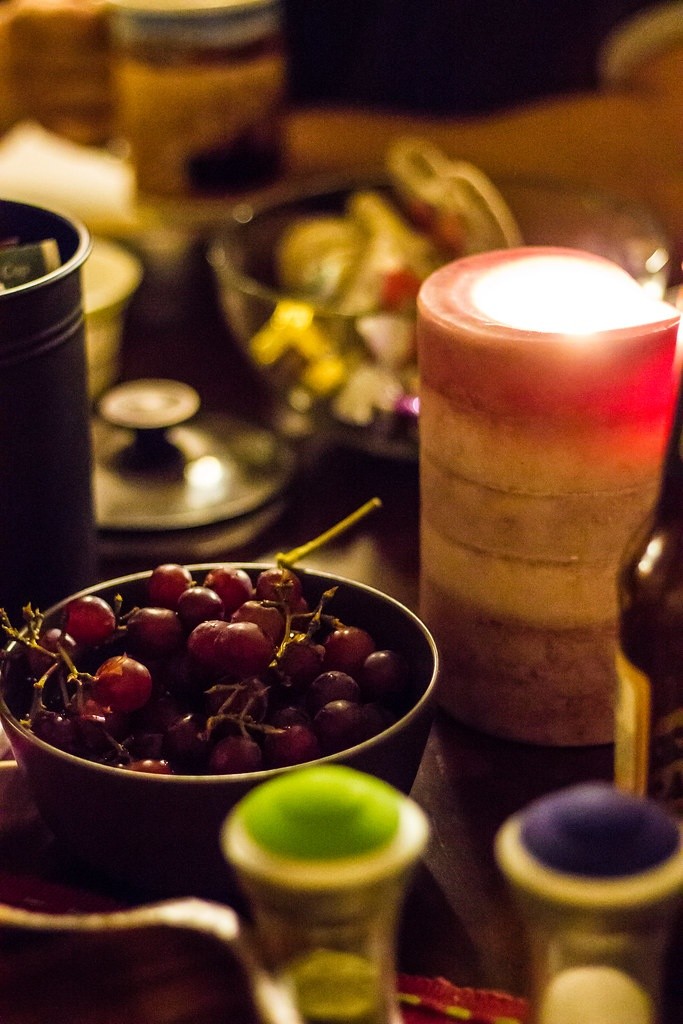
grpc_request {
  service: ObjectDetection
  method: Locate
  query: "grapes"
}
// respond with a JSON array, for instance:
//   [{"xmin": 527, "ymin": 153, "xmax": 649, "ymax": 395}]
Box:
[{"xmin": 20, "ymin": 560, "xmax": 410, "ymax": 774}]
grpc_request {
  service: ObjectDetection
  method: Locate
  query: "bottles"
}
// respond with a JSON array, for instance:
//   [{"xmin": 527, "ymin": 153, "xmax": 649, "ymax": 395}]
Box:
[
  {"xmin": 614, "ymin": 357, "xmax": 683, "ymax": 815},
  {"xmin": 495, "ymin": 785, "xmax": 683, "ymax": 1024},
  {"xmin": 113, "ymin": 4, "xmax": 284, "ymax": 219},
  {"xmin": 219, "ymin": 769, "xmax": 433, "ymax": 1021}
]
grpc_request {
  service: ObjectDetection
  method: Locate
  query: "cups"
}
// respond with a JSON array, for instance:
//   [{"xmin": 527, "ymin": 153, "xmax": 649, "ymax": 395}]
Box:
[{"xmin": 0, "ymin": 200, "xmax": 98, "ymax": 631}]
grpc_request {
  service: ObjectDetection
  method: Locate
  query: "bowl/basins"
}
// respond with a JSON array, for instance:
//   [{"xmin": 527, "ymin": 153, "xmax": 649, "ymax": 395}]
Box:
[
  {"xmin": 1, "ymin": 561, "xmax": 440, "ymax": 882},
  {"xmin": 75, "ymin": 239, "xmax": 143, "ymax": 404},
  {"xmin": 209, "ymin": 176, "xmax": 669, "ymax": 461},
  {"xmin": 78, "ymin": 379, "xmax": 297, "ymax": 566}
]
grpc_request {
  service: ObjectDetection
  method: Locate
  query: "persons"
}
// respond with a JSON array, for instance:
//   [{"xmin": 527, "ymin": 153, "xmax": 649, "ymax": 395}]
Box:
[{"xmin": 0, "ymin": 1, "xmax": 682, "ymax": 278}]
